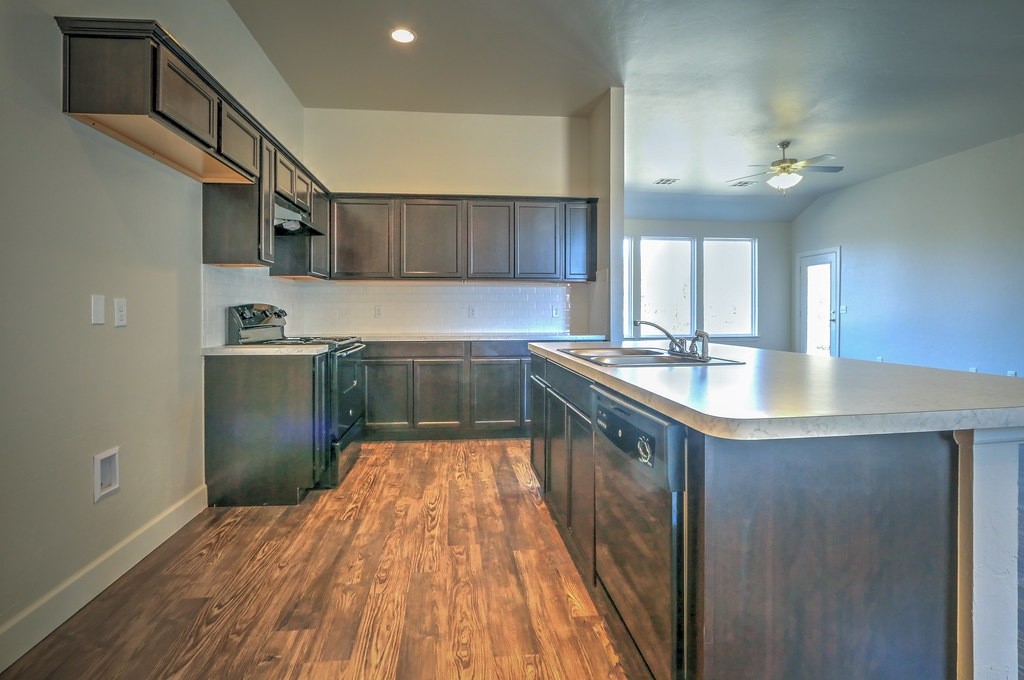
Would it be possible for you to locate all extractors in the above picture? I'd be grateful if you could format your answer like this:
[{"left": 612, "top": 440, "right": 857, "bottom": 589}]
[{"left": 274, "top": 194, "right": 325, "bottom": 238}]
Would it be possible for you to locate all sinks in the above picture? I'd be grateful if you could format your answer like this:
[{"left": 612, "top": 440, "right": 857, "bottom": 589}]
[
  {"left": 587, "top": 357, "right": 746, "bottom": 366},
  {"left": 557, "top": 348, "right": 664, "bottom": 356}
]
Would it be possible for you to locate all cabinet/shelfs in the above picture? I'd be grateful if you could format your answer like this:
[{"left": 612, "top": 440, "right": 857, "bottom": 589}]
[
  {"left": 56, "top": 17, "right": 599, "bottom": 281},
  {"left": 204, "top": 341, "right": 593, "bottom": 600}
]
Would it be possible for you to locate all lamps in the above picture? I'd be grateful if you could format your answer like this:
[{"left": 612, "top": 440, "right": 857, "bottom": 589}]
[{"left": 766, "top": 170, "right": 803, "bottom": 196}]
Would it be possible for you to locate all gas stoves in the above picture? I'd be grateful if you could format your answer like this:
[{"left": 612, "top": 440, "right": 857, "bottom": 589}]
[{"left": 226, "top": 302, "right": 364, "bottom": 353}]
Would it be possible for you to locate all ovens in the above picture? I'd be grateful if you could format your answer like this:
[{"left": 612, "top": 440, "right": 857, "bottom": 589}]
[{"left": 322, "top": 342, "right": 366, "bottom": 491}]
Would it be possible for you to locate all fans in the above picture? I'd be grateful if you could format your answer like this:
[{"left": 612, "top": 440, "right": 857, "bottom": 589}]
[{"left": 725, "top": 142, "right": 844, "bottom": 183}]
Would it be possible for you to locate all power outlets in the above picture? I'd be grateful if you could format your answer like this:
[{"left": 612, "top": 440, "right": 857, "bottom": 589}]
[
  {"left": 552, "top": 305, "right": 559, "bottom": 317},
  {"left": 374, "top": 305, "right": 382, "bottom": 318},
  {"left": 468, "top": 305, "right": 476, "bottom": 317}
]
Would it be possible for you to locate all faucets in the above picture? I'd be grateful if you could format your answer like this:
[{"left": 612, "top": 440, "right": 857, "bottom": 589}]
[
  {"left": 633, "top": 320, "right": 686, "bottom": 355},
  {"left": 695, "top": 330, "right": 710, "bottom": 360}
]
